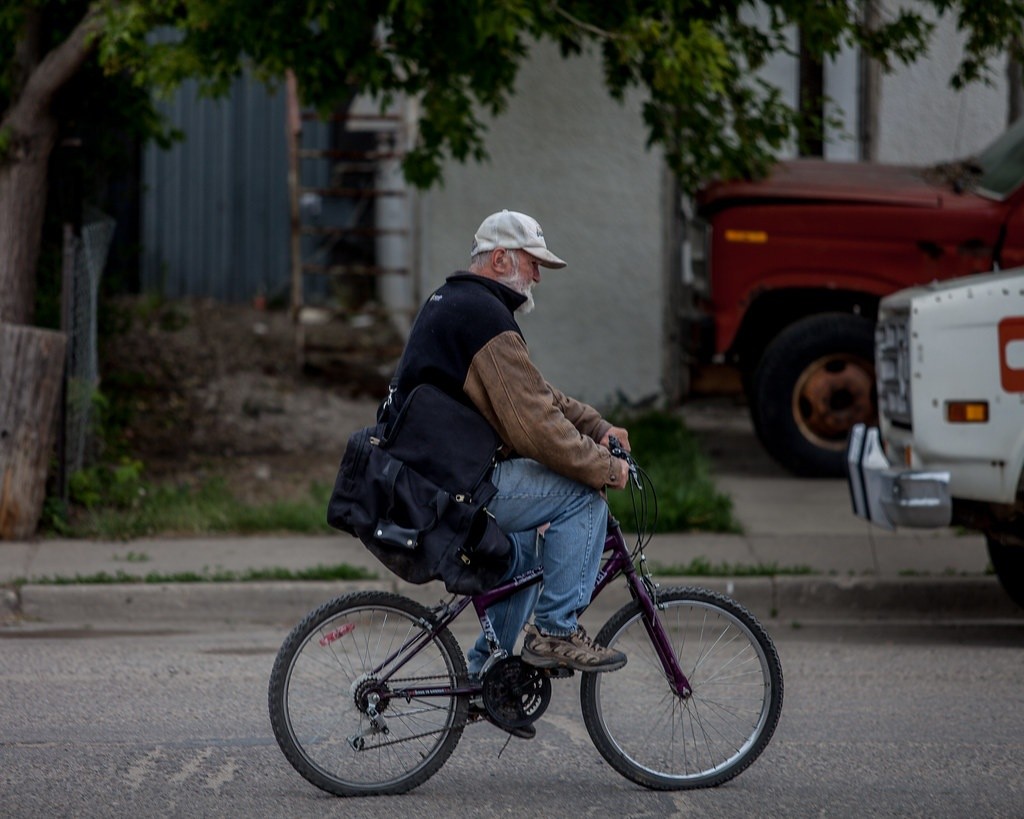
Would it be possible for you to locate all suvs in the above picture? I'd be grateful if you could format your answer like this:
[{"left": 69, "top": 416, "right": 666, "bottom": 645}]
[{"left": 693, "top": 112, "right": 1024, "bottom": 481}]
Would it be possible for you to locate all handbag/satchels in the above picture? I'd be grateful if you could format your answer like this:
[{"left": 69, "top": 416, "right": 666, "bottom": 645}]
[{"left": 326, "top": 386, "right": 513, "bottom": 595}]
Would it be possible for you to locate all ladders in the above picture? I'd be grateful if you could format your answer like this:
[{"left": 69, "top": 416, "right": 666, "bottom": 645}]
[{"left": 282, "top": 33, "right": 427, "bottom": 387}]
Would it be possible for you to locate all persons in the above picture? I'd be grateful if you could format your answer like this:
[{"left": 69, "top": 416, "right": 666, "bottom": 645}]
[{"left": 376, "top": 211, "right": 632, "bottom": 739}]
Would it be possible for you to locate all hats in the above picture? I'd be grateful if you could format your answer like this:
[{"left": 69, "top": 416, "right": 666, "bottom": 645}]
[{"left": 471, "top": 209, "right": 568, "bottom": 269}]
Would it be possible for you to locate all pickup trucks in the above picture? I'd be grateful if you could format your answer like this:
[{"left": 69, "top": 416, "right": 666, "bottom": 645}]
[{"left": 842, "top": 273, "right": 1024, "bottom": 620}]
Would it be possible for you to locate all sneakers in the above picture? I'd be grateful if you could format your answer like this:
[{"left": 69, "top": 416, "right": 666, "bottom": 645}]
[
  {"left": 520, "top": 621, "right": 626, "bottom": 671},
  {"left": 465, "top": 669, "right": 536, "bottom": 740}
]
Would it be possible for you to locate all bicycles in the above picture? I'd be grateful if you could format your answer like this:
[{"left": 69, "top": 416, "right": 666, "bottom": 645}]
[{"left": 268, "top": 435, "right": 786, "bottom": 797}]
